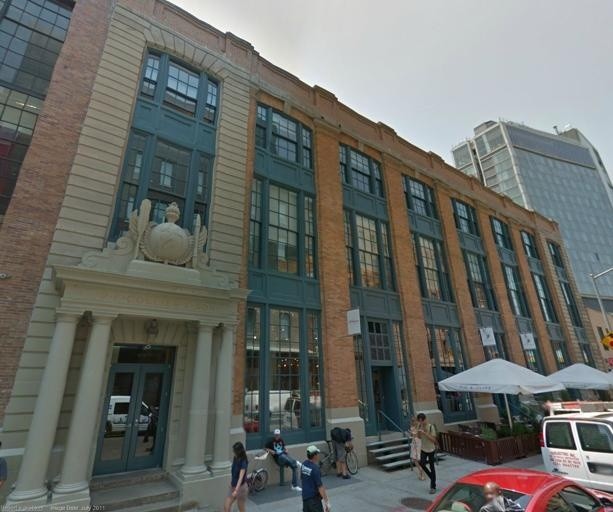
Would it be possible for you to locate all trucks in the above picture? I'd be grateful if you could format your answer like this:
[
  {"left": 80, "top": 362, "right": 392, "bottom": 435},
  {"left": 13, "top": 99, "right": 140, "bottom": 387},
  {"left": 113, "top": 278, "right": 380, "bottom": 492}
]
[
  {"left": 544, "top": 400, "right": 612, "bottom": 416},
  {"left": 243, "top": 387, "right": 291, "bottom": 421},
  {"left": 281, "top": 390, "right": 321, "bottom": 433},
  {"left": 538, "top": 400, "right": 612, "bottom": 494}
]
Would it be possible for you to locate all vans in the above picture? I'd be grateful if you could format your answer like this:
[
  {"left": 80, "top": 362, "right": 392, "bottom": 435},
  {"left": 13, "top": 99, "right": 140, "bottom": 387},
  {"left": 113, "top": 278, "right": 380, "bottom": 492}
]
[{"left": 102, "top": 394, "right": 159, "bottom": 439}]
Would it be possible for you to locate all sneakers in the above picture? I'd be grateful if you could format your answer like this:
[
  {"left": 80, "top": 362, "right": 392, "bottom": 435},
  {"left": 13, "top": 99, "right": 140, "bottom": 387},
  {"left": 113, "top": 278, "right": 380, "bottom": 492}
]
[
  {"left": 296, "top": 460, "right": 302, "bottom": 468},
  {"left": 343, "top": 475, "right": 351, "bottom": 479},
  {"left": 430, "top": 489, "right": 435, "bottom": 494},
  {"left": 337, "top": 472, "right": 342, "bottom": 477},
  {"left": 292, "top": 485, "right": 302, "bottom": 492}
]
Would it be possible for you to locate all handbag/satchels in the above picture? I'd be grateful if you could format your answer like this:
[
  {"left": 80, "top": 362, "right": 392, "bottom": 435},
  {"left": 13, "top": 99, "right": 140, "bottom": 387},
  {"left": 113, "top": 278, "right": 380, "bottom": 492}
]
[{"left": 331, "top": 427, "right": 349, "bottom": 444}]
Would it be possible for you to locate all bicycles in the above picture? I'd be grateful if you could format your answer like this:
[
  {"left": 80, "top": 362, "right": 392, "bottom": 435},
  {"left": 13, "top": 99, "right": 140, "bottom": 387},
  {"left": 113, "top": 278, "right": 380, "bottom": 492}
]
[
  {"left": 246, "top": 454, "right": 268, "bottom": 496},
  {"left": 316, "top": 437, "right": 358, "bottom": 477}
]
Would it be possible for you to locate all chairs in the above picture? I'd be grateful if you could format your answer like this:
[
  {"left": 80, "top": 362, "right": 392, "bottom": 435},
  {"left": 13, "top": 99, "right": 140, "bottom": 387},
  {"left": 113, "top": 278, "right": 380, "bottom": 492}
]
[{"left": 449, "top": 421, "right": 509, "bottom": 462}]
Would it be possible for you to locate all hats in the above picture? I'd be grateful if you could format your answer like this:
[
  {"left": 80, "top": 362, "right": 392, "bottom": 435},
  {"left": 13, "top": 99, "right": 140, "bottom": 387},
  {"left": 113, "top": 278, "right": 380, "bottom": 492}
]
[
  {"left": 274, "top": 429, "right": 280, "bottom": 435},
  {"left": 306, "top": 445, "right": 320, "bottom": 455}
]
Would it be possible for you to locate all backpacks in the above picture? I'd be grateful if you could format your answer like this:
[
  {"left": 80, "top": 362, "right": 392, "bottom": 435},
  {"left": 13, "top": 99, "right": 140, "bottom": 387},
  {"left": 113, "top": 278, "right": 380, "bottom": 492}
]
[{"left": 419, "top": 423, "right": 439, "bottom": 446}]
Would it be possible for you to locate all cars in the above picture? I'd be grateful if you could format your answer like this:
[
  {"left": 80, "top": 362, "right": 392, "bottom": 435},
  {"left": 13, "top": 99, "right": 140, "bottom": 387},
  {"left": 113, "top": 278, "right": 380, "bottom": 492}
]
[
  {"left": 424, "top": 467, "right": 612, "bottom": 511},
  {"left": 436, "top": 393, "right": 463, "bottom": 414},
  {"left": 243, "top": 416, "right": 259, "bottom": 433}
]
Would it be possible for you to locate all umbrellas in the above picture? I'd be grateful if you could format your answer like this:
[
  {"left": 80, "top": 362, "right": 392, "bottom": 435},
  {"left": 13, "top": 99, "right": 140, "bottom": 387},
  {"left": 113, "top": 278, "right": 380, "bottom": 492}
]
[
  {"left": 438, "top": 357, "right": 565, "bottom": 428},
  {"left": 547, "top": 362, "right": 613, "bottom": 390},
  {"left": 607, "top": 369, "right": 613, "bottom": 386}
]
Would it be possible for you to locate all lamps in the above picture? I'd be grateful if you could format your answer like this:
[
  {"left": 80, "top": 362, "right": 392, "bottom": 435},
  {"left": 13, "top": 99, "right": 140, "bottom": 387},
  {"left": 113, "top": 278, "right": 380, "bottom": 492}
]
[{"left": 146, "top": 319, "right": 160, "bottom": 347}]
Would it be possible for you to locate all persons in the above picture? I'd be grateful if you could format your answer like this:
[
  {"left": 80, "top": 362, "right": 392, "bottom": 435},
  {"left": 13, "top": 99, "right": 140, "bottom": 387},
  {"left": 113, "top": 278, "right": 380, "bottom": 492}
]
[
  {"left": 224, "top": 441, "right": 249, "bottom": 512},
  {"left": 332, "top": 428, "right": 353, "bottom": 480},
  {"left": 408, "top": 417, "right": 428, "bottom": 480},
  {"left": 301, "top": 446, "right": 327, "bottom": 511},
  {"left": 265, "top": 430, "right": 302, "bottom": 492},
  {"left": 417, "top": 413, "right": 437, "bottom": 494}
]
